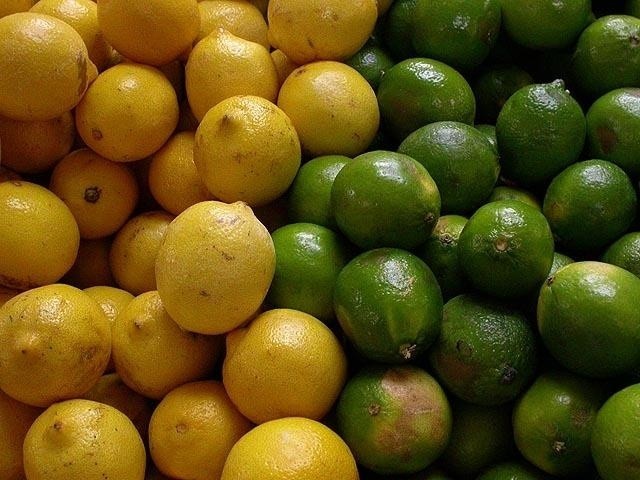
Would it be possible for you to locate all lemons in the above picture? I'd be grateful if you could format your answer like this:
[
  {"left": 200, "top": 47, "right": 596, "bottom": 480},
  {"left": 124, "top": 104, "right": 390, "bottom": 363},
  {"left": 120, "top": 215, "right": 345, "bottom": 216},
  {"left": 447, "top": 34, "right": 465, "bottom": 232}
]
[
  {"left": 269, "top": 0, "right": 640, "bottom": 479},
  {"left": 0, "top": 0, "right": 378, "bottom": 480}
]
